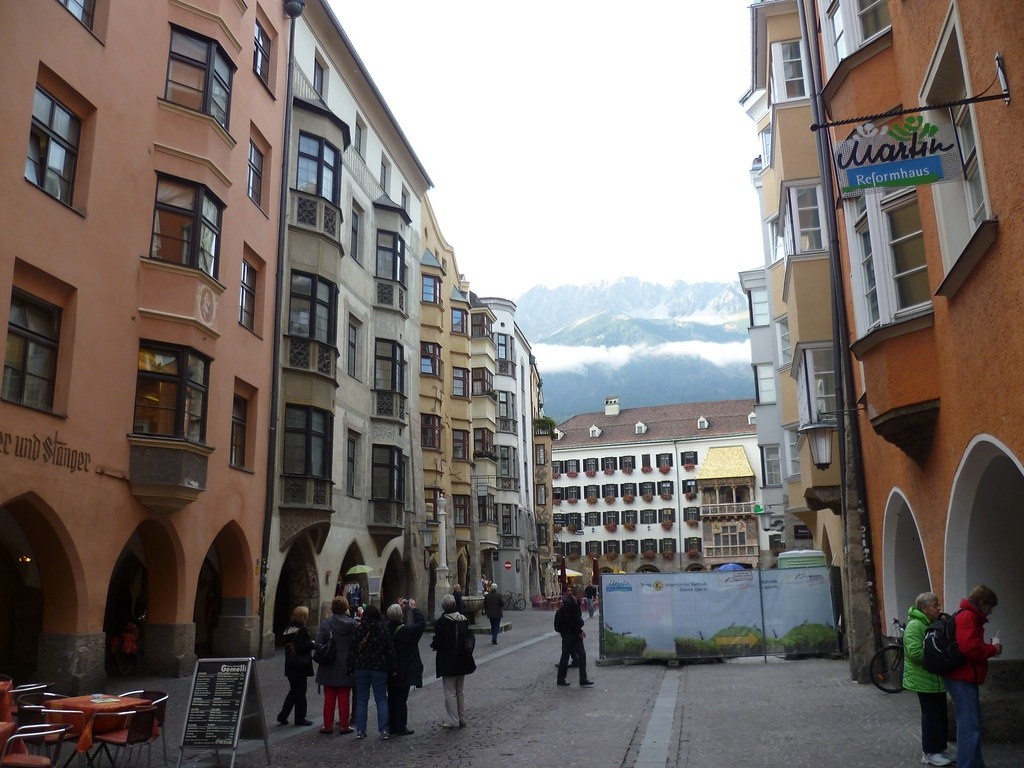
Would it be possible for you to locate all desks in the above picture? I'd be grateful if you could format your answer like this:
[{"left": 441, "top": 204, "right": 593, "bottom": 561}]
[{"left": 43, "top": 693, "right": 154, "bottom": 767}]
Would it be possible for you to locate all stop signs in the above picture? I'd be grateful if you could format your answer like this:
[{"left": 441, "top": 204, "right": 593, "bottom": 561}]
[{"left": 505, "top": 561, "right": 512, "bottom": 570}]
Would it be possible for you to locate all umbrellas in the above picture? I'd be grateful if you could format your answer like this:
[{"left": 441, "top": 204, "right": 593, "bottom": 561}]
[
  {"left": 553, "top": 568, "right": 582, "bottom": 593},
  {"left": 346, "top": 565, "right": 373, "bottom": 574},
  {"left": 719, "top": 563, "right": 744, "bottom": 570}
]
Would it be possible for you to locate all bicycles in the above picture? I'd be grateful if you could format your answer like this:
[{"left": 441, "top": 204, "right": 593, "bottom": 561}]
[
  {"left": 869, "top": 618, "right": 906, "bottom": 693},
  {"left": 503, "top": 591, "right": 526, "bottom": 611}
]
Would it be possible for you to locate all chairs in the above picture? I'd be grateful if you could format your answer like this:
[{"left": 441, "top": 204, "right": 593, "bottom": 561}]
[
  {"left": 529, "top": 593, "right": 590, "bottom": 612},
  {"left": 0, "top": 683, "right": 169, "bottom": 767}
]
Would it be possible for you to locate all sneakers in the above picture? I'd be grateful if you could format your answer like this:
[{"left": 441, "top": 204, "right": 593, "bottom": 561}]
[
  {"left": 941, "top": 750, "right": 956, "bottom": 762},
  {"left": 380, "top": 729, "right": 390, "bottom": 740},
  {"left": 356, "top": 730, "right": 367, "bottom": 739},
  {"left": 922, "top": 749, "right": 950, "bottom": 766}
]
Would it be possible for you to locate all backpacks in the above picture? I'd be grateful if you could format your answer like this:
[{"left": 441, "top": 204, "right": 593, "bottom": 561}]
[{"left": 922, "top": 607, "right": 980, "bottom": 675}]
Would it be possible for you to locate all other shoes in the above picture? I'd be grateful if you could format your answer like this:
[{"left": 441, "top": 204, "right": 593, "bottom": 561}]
[
  {"left": 389, "top": 727, "right": 414, "bottom": 735},
  {"left": 340, "top": 725, "right": 354, "bottom": 733},
  {"left": 295, "top": 719, "right": 313, "bottom": 726},
  {"left": 557, "top": 679, "right": 570, "bottom": 686},
  {"left": 555, "top": 663, "right": 559, "bottom": 667},
  {"left": 580, "top": 680, "right": 593, "bottom": 685},
  {"left": 320, "top": 726, "right": 332, "bottom": 734},
  {"left": 569, "top": 660, "right": 579, "bottom": 668},
  {"left": 277, "top": 716, "right": 288, "bottom": 724},
  {"left": 440, "top": 720, "right": 466, "bottom": 729}
]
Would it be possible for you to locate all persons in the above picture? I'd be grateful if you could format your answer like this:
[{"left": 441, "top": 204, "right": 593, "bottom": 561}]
[
  {"left": 482, "top": 573, "right": 505, "bottom": 644},
  {"left": 453, "top": 584, "right": 464, "bottom": 613},
  {"left": 555, "top": 582, "right": 594, "bottom": 685},
  {"left": 903, "top": 593, "right": 956, "bottom": 768},
  {"left": 941, "top": 585, "right": 1003, "bottom": 768},
  {"left": 276, "top": 595, "right": 426, "bottom": 740},
  {"left": 430, "top": 595, "right": 468, "bottom": 728}
]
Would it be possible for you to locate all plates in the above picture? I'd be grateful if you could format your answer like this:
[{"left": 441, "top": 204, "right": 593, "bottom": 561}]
[{"left": 90, "top": 698, "right": 105, "bottom": 703}]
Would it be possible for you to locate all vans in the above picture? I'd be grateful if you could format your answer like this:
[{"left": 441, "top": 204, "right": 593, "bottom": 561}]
[{"left": 777, "top": 551, "right": 828, "bottom": 569}]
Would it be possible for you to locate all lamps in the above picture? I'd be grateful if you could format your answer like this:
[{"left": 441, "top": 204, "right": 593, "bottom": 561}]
[{"left": 799, "top": 407, "right": 864, "bottom": 472}]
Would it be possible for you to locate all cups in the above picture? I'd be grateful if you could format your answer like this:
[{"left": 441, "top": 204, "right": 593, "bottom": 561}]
[
  {"left": 92, "top": 694, "right": 103, "bottom": 700},
  {"left": 990, "top": 637, "right": 1001, "bottom": 658}
]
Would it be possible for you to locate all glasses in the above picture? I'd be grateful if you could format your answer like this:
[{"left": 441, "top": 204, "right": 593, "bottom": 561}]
[{"left": 923, "top": 604, "right": 941, "bottom": 610}]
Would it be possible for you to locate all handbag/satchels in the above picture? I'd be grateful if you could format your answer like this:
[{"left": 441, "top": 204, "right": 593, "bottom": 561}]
[
  {"left": 110, "top": 632, "right": 139, "bottom": 655},
  {"left": 313, "top": 619, "right": 332, "bottom": 664},
  {"left": 554, "top": 609, "right": 575, "bottom": 635}
]
